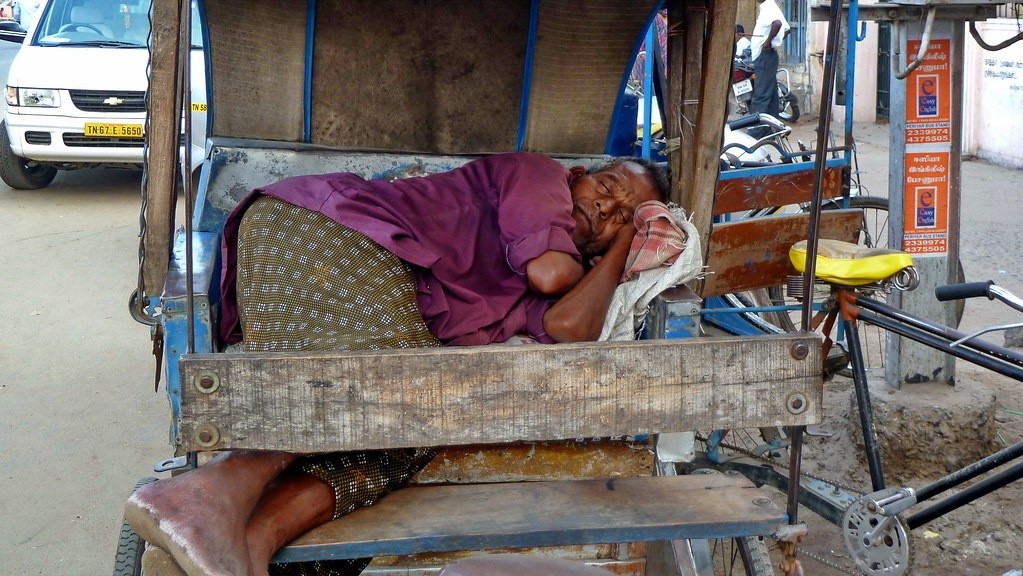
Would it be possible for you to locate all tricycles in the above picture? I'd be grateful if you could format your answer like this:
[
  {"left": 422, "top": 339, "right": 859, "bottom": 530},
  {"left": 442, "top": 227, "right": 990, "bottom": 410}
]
[
  {"left": 107, "top": 0, "right": 838, "bottom": 575},
  {"left": 618, "top": 0, "right": 1023, "bottom": 576}
]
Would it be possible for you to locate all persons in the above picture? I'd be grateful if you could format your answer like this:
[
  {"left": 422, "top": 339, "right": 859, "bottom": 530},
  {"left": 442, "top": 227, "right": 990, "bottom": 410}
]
[
  {"left": 747, "top": 0, "right": 790, "bottom": 140},
  {"left": 126, "top": 151, "right": 670, "bottom": 575},
  {"left": 735, "top": 25, "right": 750, "bottom": 61}
]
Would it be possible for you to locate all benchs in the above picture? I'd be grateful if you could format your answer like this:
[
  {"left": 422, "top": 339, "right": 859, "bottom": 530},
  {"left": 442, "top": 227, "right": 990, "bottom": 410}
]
[{"left": 161, "top": 138, "right": 705, "bottom": 464}]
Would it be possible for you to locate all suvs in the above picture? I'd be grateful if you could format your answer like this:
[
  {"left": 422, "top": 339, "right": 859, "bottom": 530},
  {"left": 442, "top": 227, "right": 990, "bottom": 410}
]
[{"left": 0, "top": 0, "right": 208, "bottom": 192}]
